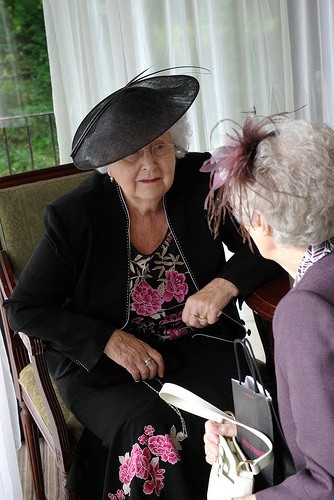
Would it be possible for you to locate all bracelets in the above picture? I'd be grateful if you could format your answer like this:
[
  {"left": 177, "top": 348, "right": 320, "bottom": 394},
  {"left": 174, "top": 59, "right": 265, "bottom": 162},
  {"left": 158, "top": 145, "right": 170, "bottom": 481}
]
[{"left": 225, "top": 411, "right": 235, "bottom": 419}]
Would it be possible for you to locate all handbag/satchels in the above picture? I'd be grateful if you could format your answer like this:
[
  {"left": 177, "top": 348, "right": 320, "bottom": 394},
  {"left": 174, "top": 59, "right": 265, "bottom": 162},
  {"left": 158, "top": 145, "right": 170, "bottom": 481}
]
[
  {"left": 159, "top": 383, "right": 274, "bottom": 500},
  {"left": 231, "top": 338, "right": 296, "bottom": 487}
]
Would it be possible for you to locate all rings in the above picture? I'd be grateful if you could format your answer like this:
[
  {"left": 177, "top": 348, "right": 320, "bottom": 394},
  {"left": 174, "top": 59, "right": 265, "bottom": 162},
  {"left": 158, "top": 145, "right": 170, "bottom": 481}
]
[
  {"left": 144, "top": 357, "right": 154, "bottom": 367},
  {"left": 199, "top": 317, "right": 207, "bottom": 324},
  {"left": 190, "top": 313, "right": 197, "bottom": 320}
]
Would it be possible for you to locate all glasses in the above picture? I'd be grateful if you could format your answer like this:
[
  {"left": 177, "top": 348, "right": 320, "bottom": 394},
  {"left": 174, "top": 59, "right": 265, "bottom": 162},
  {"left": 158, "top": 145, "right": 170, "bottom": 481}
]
[{"left": 124, "top": 144, "right": 175, "bottom": 163}]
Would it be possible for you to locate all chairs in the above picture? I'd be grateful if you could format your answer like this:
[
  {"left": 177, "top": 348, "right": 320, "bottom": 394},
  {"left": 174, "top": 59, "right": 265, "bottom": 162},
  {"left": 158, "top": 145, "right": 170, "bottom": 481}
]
[{"left": 0, "top": 158, "right": 293, "bottom": 500}]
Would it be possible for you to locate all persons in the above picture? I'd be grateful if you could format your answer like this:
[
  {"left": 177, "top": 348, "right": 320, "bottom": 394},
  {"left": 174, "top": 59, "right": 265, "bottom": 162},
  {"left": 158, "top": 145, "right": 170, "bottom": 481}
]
[
  {"left": 2, "top": 65, "right": 284, "bottom": 500},
  {"left": 200, "top": 106, "right": 334, "bottom": 500}
]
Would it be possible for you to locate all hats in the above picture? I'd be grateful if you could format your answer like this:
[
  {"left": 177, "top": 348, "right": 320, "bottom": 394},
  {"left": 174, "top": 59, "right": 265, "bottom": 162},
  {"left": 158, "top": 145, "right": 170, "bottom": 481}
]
[{"left": 69, "top": 66, "right": 211, "bottom": 170}]
